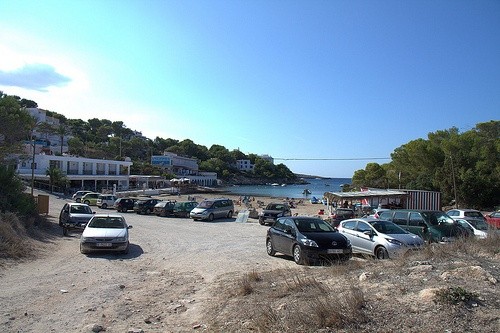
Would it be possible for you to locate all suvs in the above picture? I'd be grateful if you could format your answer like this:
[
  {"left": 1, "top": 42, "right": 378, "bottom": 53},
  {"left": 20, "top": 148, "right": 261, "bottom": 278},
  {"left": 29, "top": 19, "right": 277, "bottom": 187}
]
[
  {"left": 375, "top": 209, "right": 469, "bottom": 245},
  {"left": 190, "top": 197, "right": 235, "bottom": 222},
  {"left": 258, "top": 202, "right": 292, "bottom": 225}
]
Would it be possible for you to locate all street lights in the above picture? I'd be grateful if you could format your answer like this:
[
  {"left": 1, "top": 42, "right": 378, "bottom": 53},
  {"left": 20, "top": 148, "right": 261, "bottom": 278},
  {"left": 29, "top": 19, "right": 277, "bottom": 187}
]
[{"left": 30, "top": 138, "right": 51, "bottom": 196}]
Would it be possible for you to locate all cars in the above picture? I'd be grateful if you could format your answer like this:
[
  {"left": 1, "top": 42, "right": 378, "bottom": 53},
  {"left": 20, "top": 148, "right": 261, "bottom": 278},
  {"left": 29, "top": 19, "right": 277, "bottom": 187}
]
[
  {"left": 331, "top": 208, "right": 358, "bottom": 228},
  {"left": 437, "top": 209, "right": 500, "bottom": 241},
  {"left": 367, "top": 209, "right": 391, "bottom": 219},
  {"left": 79, "top": 214, "right": 133, "bottom": 254},
  {"left": 266, "top": 217, "right": 352, "bottom": 265},
  {"left": 333, "top": 218, "right": 425, "bottom": 260},
  {"left": 59, "top": 203, "right": 96, "bottom": 230},
  {"left": 71, "top": 190, "right": 198, "bottom": 218}
]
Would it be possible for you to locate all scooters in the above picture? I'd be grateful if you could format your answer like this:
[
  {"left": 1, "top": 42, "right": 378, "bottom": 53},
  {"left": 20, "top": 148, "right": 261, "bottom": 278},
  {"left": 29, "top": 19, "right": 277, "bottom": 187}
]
[{"left": 60, "top": 217, "right": 86, "bottom": 236}]
